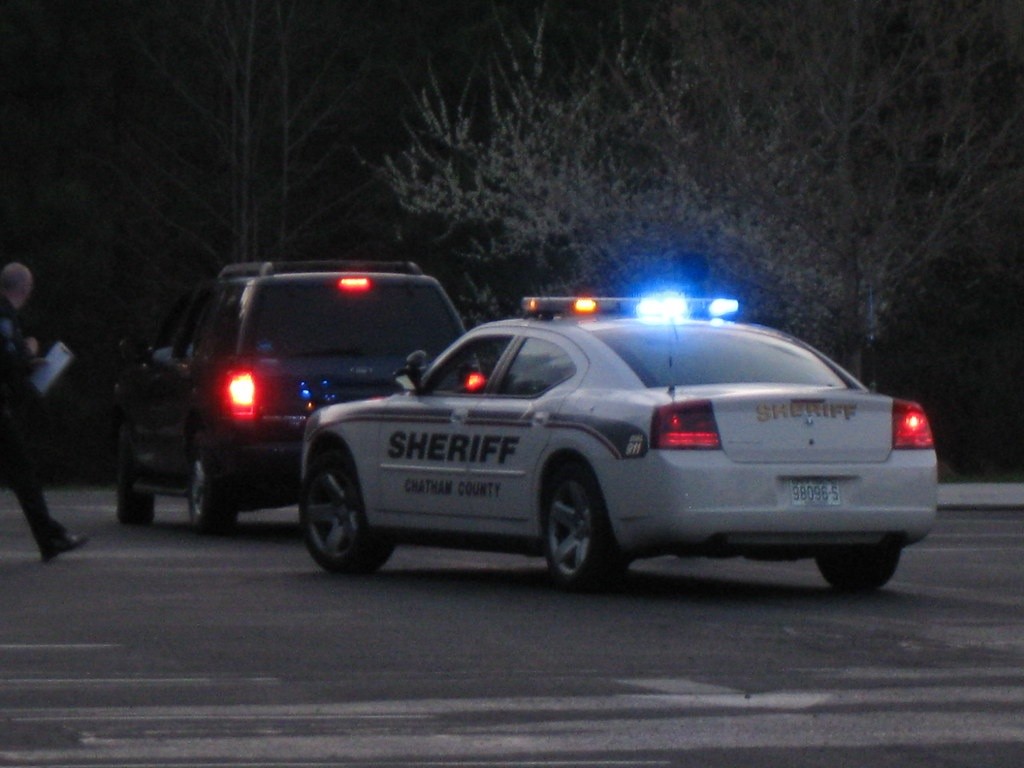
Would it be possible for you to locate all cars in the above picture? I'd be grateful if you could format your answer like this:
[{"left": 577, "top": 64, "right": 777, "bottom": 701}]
[{"left": 300, "top": 295, "right": 938, "bottom": 591}]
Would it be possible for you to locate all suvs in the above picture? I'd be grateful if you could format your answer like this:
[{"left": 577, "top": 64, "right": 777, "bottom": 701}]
[{"left": 115, "top": 261, "right": 484, "bottom": 533}]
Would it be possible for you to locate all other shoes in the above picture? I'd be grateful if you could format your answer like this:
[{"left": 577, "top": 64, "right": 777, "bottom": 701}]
[{"left": 40, "top": 530, "right": 88, "bottom": 561}]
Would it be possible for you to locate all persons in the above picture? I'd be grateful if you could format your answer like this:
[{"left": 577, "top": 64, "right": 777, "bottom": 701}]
[{"left": 0, "top": 262, "right": 88, "bottom": 562}]
